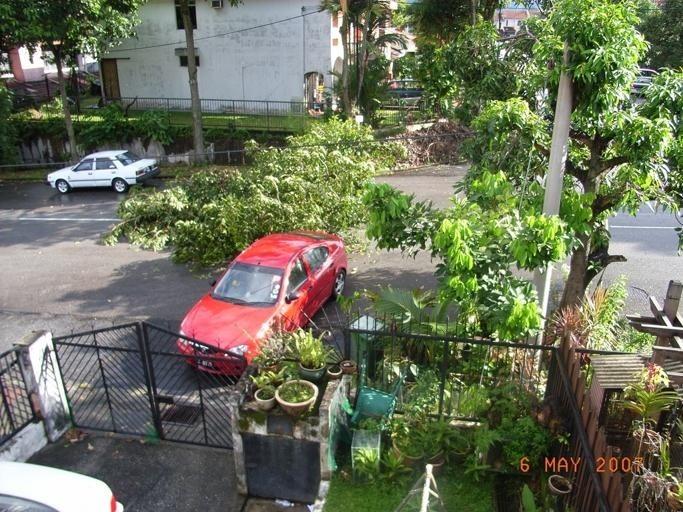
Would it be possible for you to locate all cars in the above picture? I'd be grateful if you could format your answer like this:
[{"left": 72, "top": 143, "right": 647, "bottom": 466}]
[
  {"left": 75, "top": 61, "right": 101, "bottom": 96},
  {"left": 47, "top": 150, "right": 161, "bottom": 194},
  {"left": 0, "top": 461, "right": 124, "bottom": 512},
  {"left": 176, "top": 230, "right": 347, "bottom": 377}
]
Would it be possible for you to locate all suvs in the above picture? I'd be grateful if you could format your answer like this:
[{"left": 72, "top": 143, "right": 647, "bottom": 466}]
[{"left": 368, "top": 79, "right": 431, "bottom": 111}]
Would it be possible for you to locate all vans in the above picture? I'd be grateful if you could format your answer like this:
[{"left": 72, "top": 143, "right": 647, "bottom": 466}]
[{"left": 630, "top": 68, "right": 661, "bottom": 94}]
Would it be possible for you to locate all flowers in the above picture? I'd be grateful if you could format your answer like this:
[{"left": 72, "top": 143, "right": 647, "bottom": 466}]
[{"left": 620, "top": 362, "right": 678, "bottom": 437}]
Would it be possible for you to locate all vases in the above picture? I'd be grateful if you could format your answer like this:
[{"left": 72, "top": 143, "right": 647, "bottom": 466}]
[{"left": 631, "top": 429, "right": 662, "bottom": 455}]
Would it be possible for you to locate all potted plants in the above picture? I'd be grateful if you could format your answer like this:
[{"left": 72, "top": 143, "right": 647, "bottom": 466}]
[
  {"left": 247, "top": 322, "right": 358, "bottom": 421},
  {"left": 387, "top": 408, "right": 576, "bottom": 500}
]
